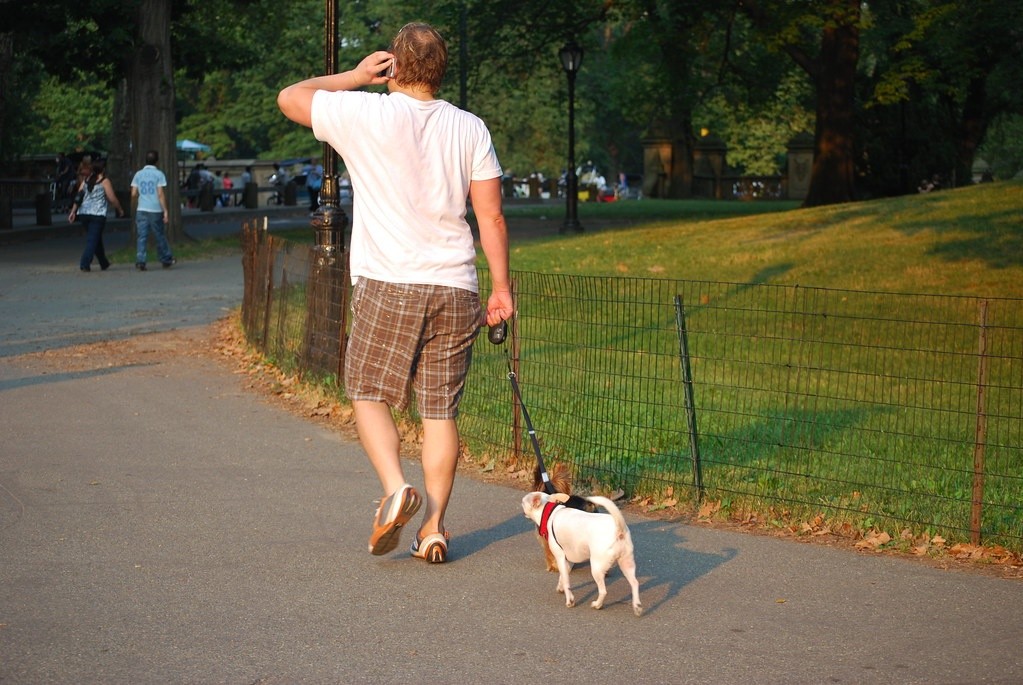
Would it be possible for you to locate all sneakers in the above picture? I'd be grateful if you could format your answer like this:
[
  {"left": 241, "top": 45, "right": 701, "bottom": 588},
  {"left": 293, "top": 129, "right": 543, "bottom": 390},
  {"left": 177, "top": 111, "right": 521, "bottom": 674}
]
[
  {"left": 368, "top": 483, "right": 423, "bottom": 556},
  {"left": 410, "top": 527, "right": 450, "bottom": 563}
]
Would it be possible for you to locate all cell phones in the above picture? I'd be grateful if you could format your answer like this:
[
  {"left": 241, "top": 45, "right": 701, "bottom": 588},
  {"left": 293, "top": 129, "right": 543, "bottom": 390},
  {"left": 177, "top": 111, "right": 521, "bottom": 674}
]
[{"left": 380, "top": 58, "right": 396, "bottom": 77}]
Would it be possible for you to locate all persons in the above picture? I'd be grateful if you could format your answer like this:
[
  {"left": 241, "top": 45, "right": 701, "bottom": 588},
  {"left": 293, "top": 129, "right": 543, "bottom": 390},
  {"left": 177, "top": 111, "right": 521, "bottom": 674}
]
[
  {"left": 181, "top": 162, "right": 233, "bottom": 207},
  {"left": 613, "top": 170, "right": 627, "bottom": 200},
  {"left": 236, "top": 166, "right": 252, "bottom": 207},
  {"left": 75, "top": 155, "right": 92, "bottom": 190},
  {"left": 278, "top": 22, "right": 513, "bottom": 563},
  {"left": 593, "top": 172, "right": 607, "bottom": 203},
  {"left": 273, "top": 163, "right": 297, "bottom": 206},
  {"left": 130, "top": 150, "right": 176, "bottom": 270},
  {"left": 305, "top": 158, "right": 323, "bottom": 212},
  {"left": 69, "top": 158, "right": 125, "bottom": 272}
]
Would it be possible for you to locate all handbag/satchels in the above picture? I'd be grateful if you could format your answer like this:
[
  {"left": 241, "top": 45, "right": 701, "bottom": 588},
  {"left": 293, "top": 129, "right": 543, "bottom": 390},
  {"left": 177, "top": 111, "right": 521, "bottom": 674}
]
[{"left": 74, "top": 190, "right": 85, "bottom": 207}]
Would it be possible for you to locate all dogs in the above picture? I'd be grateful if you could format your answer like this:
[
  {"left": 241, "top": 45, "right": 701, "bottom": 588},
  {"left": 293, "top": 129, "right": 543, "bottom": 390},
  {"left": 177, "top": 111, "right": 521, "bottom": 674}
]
[
  {"left": 534, "top": 463, "right": 600, "bottom": 572},
  {"left": 521, "top": 491, "right": 643, "bottom": 617}
]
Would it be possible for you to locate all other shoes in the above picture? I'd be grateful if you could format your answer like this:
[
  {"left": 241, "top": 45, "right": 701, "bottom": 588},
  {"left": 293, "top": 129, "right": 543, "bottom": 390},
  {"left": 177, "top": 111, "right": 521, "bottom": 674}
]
[
  {"left": 80, "top": 266, "right": 90, "bottom": 272},
  {"left": 162, "top": 258, "right": 176, "bottom": 268},
  {"left": 101, "top": 262, "right": 112, "bottom": 270},
  {"left": 136, "top": 262, "right": 147, "bottom": 271}
]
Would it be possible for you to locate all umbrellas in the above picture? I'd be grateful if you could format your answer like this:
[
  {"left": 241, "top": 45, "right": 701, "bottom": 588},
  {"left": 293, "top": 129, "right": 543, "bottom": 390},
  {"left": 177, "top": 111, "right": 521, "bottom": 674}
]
[{"left": 176, "top": 138, "right": 211, "bottom": 182}]
